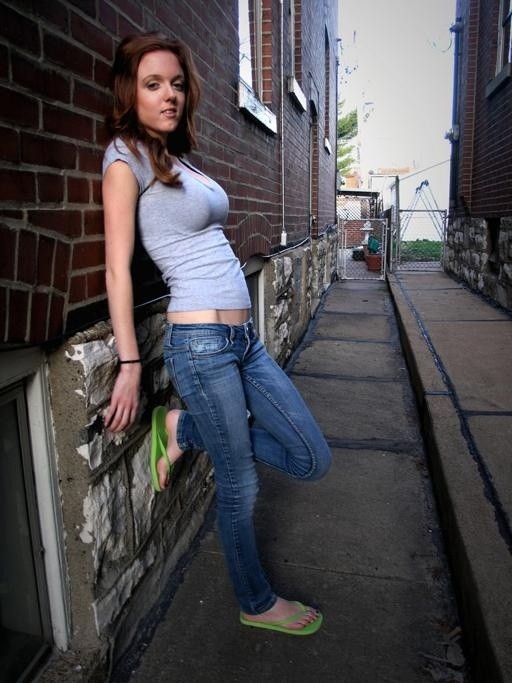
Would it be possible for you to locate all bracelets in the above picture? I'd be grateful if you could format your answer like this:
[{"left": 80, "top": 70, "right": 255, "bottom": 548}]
[{"left": 116, "top": 358, "right": 144, "bottom": 364}]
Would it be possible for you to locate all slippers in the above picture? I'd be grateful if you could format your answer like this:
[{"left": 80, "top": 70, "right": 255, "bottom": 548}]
[
  {"left": 151, "top": 406, "right": 177, "bottom": 493},
  {"left": 240, "top": 600, "right": 323, "bottom": 635}
]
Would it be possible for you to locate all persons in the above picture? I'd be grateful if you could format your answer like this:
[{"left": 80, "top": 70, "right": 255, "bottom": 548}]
[{"left": 101, "top": 29, "right": 333, "bottom": 637}]
